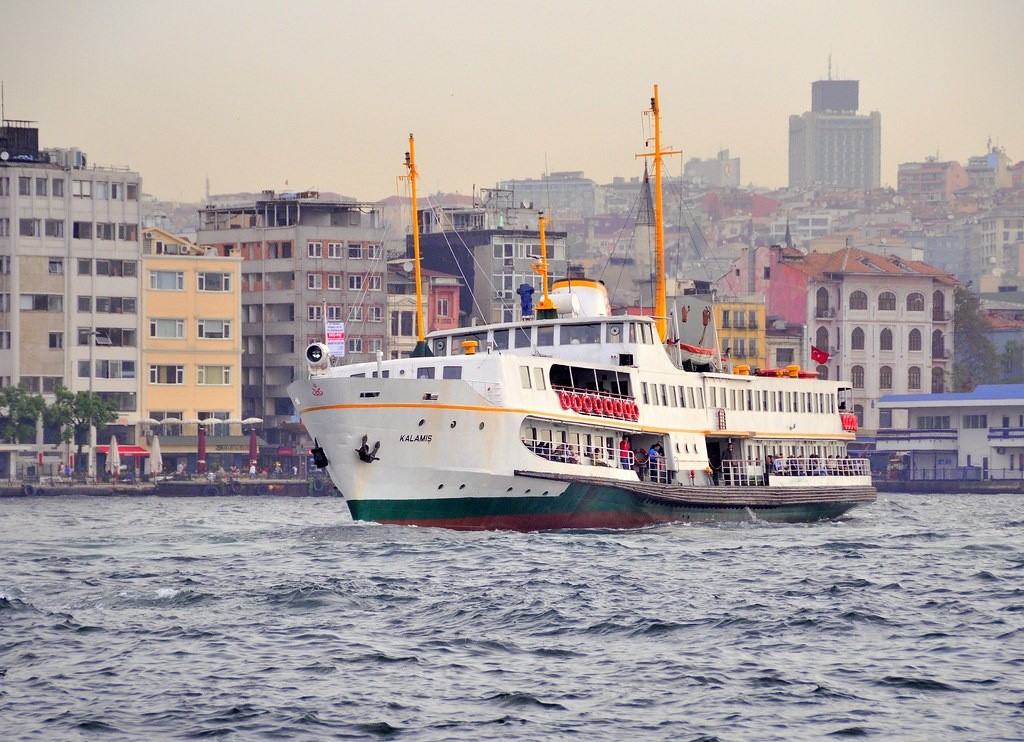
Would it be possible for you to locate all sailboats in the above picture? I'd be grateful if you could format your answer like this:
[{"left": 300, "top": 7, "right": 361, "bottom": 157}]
[{"left": 287, "top": 80, "right": 878, "bottom": 531}]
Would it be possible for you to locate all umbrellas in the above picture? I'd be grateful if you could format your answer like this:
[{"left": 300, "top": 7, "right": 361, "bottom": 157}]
[
  {"left": 196, "top": 426, "right": 206, "bottom": 478},
  {"left": 248, "top": 427, "right": 258, "bottom": 469},
  {"left": 149, "top": 433, "right": 163, "bottom": 486},
  {"left": 105, "top": 436, "right": 121, "bottom": 485},
  {"left": 138, "top": 417, "right": 264, "bottom": 437}
]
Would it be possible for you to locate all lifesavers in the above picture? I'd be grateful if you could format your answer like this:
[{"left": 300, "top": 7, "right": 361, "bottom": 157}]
[
  {"left": 843, "top": 415, "right": 858, "bottom": 430},
  {"left": 560, "top": 393, "right": 571, "bottom": 410},
  {"left": 592, "top": 397, "right": 604, "bottom": 414},
  {"left": 581, "top": 395, "right": 593, "bottom": 412},
  {"left": 570, "top": 394, "right": 583, "bottom": 411},
  {"left": 630, "top": 402, "right": 640, "bottom": 419},
  {"left": 603, "top": 399, "right": 614, "bottom": 415},
  {"left": 622, "top": 402, "right": 631, "bottom": 418},
  {"left": 612, "top": 401, "right": 623, "bottom": 417}
]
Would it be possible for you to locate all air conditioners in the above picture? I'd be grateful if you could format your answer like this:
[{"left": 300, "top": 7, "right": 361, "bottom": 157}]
[
  {"left": 494, "top": 290, "right": 505, "bottom": 298},
  {"left": 180, "top": 245, "right": 188, "bottom": 253},
  {"left": 823, "top": 311, "right": 831, "bottom": 318},
  {"left": 145, "top": 232, "right": 153, "bottom": 239}
]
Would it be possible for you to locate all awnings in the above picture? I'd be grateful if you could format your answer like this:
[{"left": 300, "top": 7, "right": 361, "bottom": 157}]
[{"left": 96, "top": 446, "right": 149, "bottom": 458}]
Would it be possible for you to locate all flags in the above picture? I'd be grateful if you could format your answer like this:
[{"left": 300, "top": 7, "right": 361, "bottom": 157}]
[{"left": 811, "top": 345, "right": 829, "bottom": 364}]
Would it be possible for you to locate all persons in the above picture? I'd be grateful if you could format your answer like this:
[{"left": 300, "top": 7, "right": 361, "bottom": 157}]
[
  {"left": 523, "top": 436, "right": 908, "bottom": 481},
  {"left": 176, "top": 460, "right": 326, "bottom": 481},
  {"left": 58, "top": 462, "right": 141, "bottom": 487}
]
